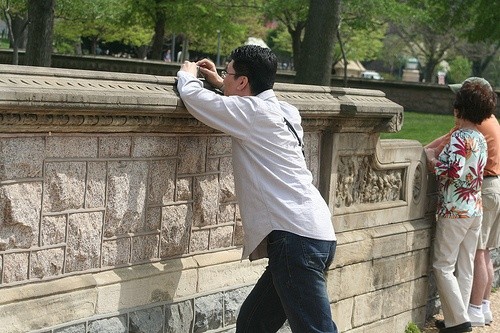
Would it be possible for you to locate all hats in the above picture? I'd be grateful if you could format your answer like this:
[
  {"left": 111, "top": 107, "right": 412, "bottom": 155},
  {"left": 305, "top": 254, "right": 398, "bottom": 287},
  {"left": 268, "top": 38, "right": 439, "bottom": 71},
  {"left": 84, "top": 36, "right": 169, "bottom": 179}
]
[{"left": 448, "top": 77, "right": 493, "bottom": 96}]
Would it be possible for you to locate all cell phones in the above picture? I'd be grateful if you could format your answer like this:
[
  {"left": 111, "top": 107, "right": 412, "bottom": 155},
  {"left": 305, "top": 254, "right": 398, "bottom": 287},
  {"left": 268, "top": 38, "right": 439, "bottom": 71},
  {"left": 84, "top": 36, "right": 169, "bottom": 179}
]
[{"left": 195, "top": 65, "right": 206, "bottom": 78}]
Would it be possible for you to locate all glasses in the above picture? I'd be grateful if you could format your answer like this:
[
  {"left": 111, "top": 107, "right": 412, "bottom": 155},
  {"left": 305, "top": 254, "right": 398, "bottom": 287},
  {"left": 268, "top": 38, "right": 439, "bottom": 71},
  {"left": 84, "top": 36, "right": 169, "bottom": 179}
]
[{"left": 220, "top": 70, "right": 240, "bottom": 79}]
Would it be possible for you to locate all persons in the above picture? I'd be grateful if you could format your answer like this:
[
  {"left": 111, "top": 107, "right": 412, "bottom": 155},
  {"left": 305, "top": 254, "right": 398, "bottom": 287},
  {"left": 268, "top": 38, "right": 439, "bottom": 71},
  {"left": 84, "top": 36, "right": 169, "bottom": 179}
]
[
  {"left": 422, "top": 76, "right": 499, "bottom": 327},
  {"left": 176, "top": 44, "right": 340, "bottom": 333},
  {"left": 423, "top": 82, "right": 488, "bottom": 333}
]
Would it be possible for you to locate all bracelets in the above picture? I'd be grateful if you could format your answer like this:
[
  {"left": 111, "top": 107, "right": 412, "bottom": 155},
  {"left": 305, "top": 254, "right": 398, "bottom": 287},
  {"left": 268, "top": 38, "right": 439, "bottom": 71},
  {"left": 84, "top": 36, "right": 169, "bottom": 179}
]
[{"left": 429, "top": 157, "right": 435, "bottom": 166}]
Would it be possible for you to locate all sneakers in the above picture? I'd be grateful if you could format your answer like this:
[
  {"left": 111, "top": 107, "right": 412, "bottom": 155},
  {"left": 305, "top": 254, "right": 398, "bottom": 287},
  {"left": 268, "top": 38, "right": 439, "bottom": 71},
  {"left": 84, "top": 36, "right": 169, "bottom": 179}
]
[
  {"left": 440, "top": 322, "right": 472, "bottom": 332},
  {"left": 482, "top": 311, "right": 492, "bottom": 325},
  {"left": 435, "top": 320, "right": 445, "bottom": 328},
  {"left": 467, "top": 314, "right": 486, "bottom": 326}
]
